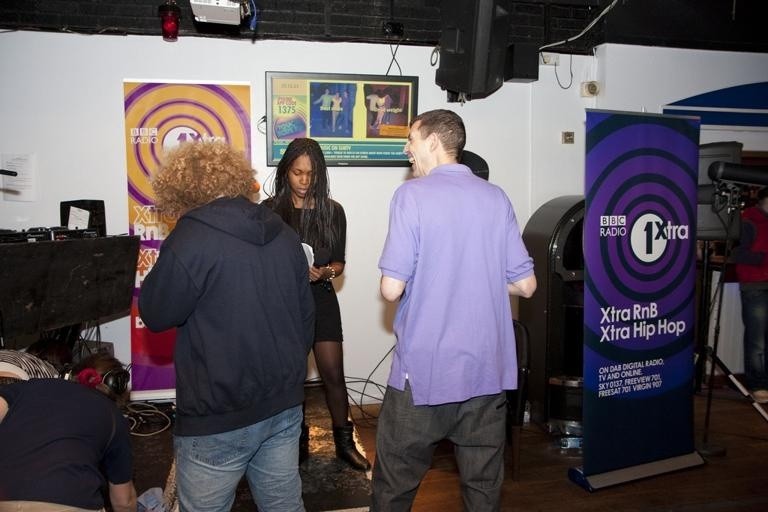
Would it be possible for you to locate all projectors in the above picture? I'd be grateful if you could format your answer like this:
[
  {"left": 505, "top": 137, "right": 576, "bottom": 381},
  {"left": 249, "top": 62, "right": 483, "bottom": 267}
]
[{"left": 189, "top": 0, "right": 251, "bottom": 25}]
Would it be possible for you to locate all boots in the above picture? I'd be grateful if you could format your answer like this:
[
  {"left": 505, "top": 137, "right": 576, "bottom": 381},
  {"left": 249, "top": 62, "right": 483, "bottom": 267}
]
[
  {"left": 299, "top": 424, "right": 310, "bottom": 466},
  {"left": 332, "top": 421, "right": 371, "bottom": 473}
]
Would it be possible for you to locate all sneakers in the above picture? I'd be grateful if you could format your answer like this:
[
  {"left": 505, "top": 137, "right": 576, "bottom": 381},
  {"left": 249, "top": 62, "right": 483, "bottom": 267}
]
[{"left": 748, "top": 387, "right": 768, "bottom": 404}]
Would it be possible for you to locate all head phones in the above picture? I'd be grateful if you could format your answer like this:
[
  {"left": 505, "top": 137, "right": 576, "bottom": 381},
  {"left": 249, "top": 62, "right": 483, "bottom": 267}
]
[{"left": 60, "top": 354, "right": 135, "bottom": 397}]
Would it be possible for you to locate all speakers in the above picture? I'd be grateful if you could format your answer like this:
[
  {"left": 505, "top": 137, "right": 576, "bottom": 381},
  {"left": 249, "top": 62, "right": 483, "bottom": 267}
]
[{"left": 695, "top": 142, "right": 742, "bottom": 240}]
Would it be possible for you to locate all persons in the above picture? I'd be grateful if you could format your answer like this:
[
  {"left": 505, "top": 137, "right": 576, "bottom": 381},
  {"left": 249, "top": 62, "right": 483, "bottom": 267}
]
[
  {"left": 313, "top": 86, "right": 331, "bottom": 129},
  {"left": 339, "top": 89, "right": 351, "bottom": 133},
  {"left": 365, "top": 89, "right": 388, "bottom": 128},
  {"left": 376, "top": 87, "right": 396, "bottom": 125},
  {"left": 258, "top": 137, "right": 371, "bottom": 473},
  {"left": 367, "top": 107, "right": 540, "bottom": 512},
  {"left": 0, "top": 353, "right": 143, "bottom": 512},
  {"left": 330, "top": 90, "right": 341, "bottom": 132},
  {"left": 733, "top": 185, "right": 767, "bottom": 404},
  {"left": 137, "top": 136, "right": 317, "bottom": 512}
]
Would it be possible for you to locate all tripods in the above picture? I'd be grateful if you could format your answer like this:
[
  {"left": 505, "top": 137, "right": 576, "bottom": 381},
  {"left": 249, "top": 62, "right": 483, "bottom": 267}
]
[{"left": 695, "top": 241, "right": 768, "bottom": 426}]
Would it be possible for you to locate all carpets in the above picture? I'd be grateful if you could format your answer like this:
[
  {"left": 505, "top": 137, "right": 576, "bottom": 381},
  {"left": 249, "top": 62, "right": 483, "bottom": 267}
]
[{"left": 121, "top": 381, "right": 375, "bottom": 510}]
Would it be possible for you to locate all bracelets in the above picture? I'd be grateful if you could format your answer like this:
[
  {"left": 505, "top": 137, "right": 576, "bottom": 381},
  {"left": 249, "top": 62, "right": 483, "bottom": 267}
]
[{"left": 321, "top": 265, "right": 335, "bottom": 283}]
